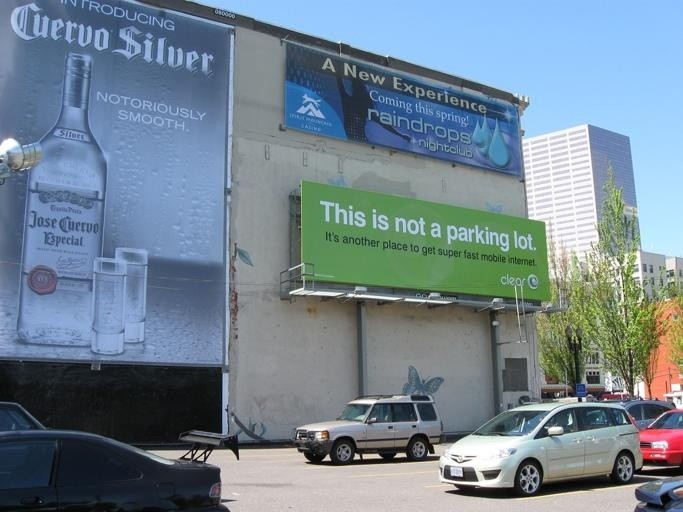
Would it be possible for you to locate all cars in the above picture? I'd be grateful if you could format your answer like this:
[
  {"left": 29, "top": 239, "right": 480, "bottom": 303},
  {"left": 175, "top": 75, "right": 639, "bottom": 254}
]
[{"left": 0, "top": 400, "right": 239, "bottom": 511}]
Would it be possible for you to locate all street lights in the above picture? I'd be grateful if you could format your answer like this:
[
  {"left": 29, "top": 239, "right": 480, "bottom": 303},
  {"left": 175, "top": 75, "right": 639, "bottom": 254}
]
[{"left": 564, "top": 326, "right": 583, "bottom": 403}]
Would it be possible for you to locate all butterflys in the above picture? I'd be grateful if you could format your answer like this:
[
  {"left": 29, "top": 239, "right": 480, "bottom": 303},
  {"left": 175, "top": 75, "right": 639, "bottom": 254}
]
[{"left": 401, "top": 365, "right": 444, "bottom": 394}]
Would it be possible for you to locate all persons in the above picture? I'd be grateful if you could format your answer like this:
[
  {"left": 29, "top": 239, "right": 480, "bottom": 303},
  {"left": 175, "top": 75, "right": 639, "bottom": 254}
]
[{"left": 337, "top": 75, "right": 411, "bottom": 143}]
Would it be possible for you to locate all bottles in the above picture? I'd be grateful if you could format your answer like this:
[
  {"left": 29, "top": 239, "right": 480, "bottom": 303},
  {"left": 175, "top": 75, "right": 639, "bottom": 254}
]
[{"left": 14, "top": 53, "right": 108, "bottom": 351}]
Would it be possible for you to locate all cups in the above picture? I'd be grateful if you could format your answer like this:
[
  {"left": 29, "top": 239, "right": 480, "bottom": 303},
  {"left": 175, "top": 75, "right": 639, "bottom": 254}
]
[{"left": 90, "top": 245, "right": 148, "bottom": 357}]
